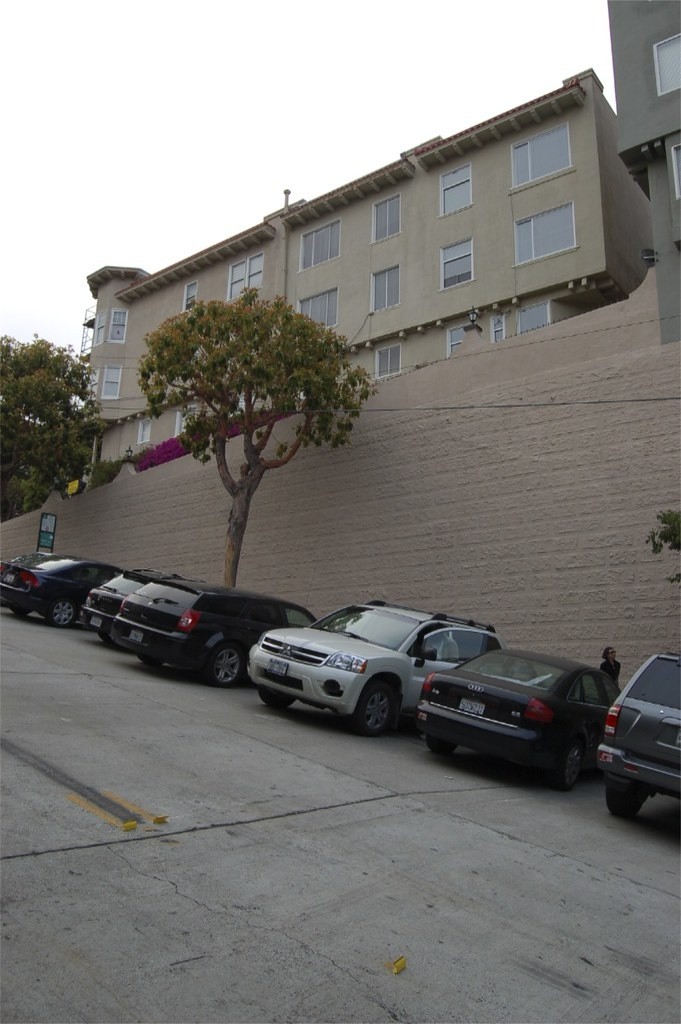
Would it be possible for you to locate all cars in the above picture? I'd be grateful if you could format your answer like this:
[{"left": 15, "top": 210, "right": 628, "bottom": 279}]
[
  {"left": 111, "top": 579, "right": 318, "bottom": 690},
  {"left": 414, "top": 648, "right": 622, "bottom": 793},
  {"left": 0, "top": 551, "right": 125, "bottom": 629},
  {"left": 80, "top": 567, "right": 188, "bottom": 644}
]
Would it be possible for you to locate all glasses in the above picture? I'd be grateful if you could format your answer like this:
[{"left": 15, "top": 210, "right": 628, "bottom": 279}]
[{"left": 609, "top": 651, "right": 616, "bottom": 654}]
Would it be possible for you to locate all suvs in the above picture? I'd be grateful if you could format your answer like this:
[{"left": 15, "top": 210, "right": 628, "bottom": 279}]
[
  {"left": 246, "top": 599, "right": 508, "bottom": 739},
  {"left": 596, "top": 651, "right": 680, "bottom": 819}
]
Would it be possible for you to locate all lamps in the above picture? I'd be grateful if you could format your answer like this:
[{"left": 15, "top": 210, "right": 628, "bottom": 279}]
[
  {"left": 126, "top": 444, "right": 133, "bottom": 459},
  {"left": 468, "top": 307, "right": 480, "bottom": 326}
]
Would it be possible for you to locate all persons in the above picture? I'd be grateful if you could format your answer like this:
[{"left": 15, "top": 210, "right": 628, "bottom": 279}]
[
  {"left": 599, "top": 647, "right": 621, "bottom": 682},
  {"left": 426, "top": 631, "right": 459, "bottom": 660}
]
